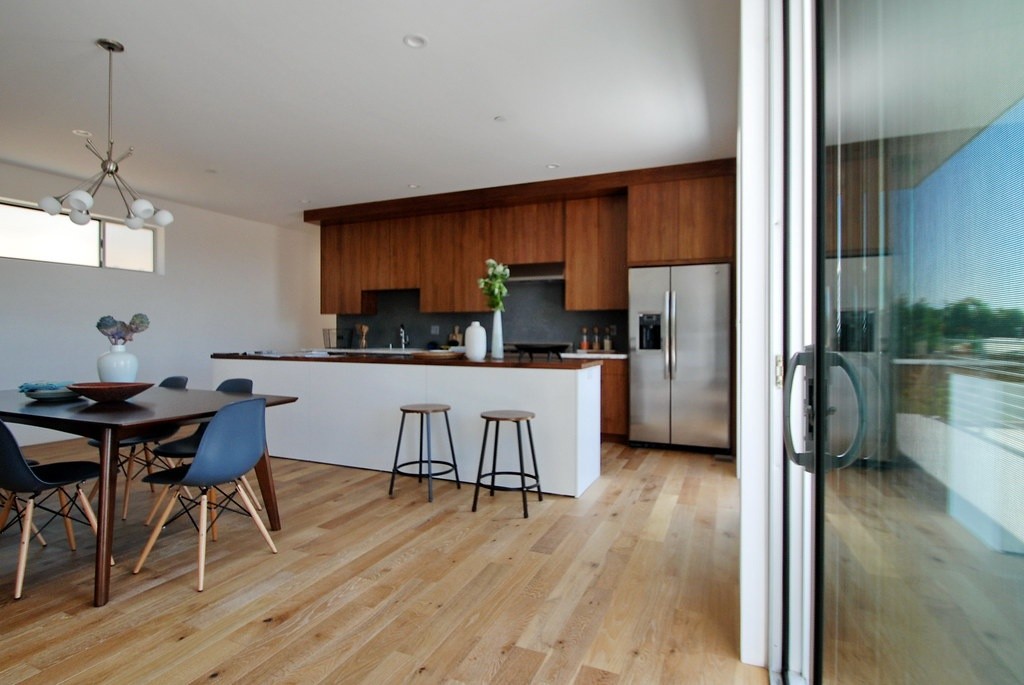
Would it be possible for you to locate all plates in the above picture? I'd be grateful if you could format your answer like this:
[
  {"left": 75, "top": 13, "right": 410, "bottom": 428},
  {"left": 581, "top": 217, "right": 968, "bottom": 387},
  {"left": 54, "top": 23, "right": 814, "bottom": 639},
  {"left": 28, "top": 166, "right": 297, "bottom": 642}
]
[
  {"left": 414, "top": 351, "right": 460, "bottom": 361},
  {"left": 24, "top": 387, "right": 80, "bottom": 402}
]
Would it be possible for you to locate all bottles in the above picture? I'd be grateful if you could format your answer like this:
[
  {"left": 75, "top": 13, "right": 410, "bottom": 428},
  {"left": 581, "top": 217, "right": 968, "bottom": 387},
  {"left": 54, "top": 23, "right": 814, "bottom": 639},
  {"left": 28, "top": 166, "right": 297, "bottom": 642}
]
[
  {"left": 580, "top": 327, "right": 590, "bottom": 350},
  {"left": 603, "top": 328, "right": 612, "bottom": 351},
  {"left": 359, "top": 335, "right": 367, "bottom": 348},
  {"left": 592, "top": 327, "right": 600, "bottom": 350},
  {"left": 464, "top": 320, "right": 486, "bottom": 360}
]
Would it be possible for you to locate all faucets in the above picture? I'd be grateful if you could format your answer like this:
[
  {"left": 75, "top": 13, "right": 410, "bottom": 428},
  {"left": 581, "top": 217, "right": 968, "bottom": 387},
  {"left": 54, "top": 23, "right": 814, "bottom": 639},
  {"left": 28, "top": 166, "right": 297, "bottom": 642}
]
[{"left": 399, "top": 324, "right": 410, "bottom": 348}]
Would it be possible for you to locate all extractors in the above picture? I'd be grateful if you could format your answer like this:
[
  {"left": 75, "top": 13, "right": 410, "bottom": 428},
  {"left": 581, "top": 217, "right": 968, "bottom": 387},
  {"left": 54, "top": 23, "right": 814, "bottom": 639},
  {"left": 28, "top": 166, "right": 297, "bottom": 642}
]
[{"left": 496, "top": 262, "right": 565, "bottom": 280}]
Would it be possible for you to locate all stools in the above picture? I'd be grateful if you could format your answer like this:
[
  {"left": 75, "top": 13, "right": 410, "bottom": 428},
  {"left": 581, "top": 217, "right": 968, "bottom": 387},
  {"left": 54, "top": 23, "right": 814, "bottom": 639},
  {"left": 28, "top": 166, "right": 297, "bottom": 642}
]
[
  {"left": 473, "top": 409, "right": 543, "bottom": 518},
  {"left": 388, "top": 403, "right": 461, "bottom": 502}
]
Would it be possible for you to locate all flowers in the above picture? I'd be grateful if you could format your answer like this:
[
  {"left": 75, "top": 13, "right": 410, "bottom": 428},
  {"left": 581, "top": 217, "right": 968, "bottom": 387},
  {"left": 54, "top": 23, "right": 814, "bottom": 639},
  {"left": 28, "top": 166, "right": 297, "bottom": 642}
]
[{"left": 477, "top": 259, "right": 510, "bottom": 311}]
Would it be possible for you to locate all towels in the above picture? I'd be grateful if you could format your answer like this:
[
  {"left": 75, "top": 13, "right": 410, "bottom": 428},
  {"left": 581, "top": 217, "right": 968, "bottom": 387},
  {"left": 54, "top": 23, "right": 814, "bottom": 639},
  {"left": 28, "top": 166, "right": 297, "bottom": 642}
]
[{"left": 18, "top": 379, "right": 74, "bottom": 393}]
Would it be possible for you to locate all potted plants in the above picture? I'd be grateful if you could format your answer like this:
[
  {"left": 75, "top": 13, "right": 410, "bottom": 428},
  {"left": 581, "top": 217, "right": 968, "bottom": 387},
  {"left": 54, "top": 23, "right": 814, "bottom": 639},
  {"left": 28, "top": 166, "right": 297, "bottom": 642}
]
[{"left": 96, "top": 313, "right": 150, "bottom": 383}]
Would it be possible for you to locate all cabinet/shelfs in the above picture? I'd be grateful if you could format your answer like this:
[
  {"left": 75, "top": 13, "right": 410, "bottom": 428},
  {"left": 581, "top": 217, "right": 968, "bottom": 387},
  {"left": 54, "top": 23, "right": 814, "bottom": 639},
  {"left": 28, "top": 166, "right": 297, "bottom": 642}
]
[{"left": 320, "top": 176, "right": 735, "bottom": 440}]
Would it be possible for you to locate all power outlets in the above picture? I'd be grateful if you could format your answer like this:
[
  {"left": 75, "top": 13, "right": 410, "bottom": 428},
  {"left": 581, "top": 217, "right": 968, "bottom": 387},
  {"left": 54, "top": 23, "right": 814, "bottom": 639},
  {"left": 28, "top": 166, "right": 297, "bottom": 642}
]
[
  {"left": 610, "top": 325, "right": 617, "bottom": 336},
  {"left": 431, "top": 325, "right": 439, "bottom": 335}
]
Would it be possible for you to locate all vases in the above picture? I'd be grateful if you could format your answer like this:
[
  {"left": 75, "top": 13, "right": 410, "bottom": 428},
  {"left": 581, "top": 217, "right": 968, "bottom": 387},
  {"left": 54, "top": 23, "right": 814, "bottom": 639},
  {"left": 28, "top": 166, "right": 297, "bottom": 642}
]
[
  {"left": 491, "top": 308, "right": 505, "bottom": 359},
  {"left": 465, "top": 321, "right": 487, "bottom": 360}
]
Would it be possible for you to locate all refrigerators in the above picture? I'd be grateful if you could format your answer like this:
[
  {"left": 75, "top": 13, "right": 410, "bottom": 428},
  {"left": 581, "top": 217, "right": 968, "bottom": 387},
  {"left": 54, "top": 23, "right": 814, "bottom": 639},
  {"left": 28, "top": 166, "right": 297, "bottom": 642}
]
[{"left": 628, "top": 263, "right": 731, "bottom": 449}]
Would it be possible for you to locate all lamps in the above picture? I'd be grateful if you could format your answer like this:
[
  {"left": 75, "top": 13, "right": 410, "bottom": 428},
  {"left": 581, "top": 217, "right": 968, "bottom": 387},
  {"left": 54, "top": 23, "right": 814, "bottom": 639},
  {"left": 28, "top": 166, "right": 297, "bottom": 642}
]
[{"left": 36, "top": 37, "right": 175, "bottom": 230}]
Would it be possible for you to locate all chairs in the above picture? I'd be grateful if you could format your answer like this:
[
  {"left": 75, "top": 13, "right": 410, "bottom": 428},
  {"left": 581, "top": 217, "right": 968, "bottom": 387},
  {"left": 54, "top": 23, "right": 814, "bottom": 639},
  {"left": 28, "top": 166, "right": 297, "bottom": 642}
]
[
  {"left": 145, "top": 379, "right": 262, "bottom": 541},
  {"left": 0, "top": 459, "right": 48, "bottom": 548},
  {"left": 131, "top": 398, "right": 279, "bottom": 591},
  {"left": 87, "top": 376, "right": 193, "bottom": 521},
  {"left": 0, "top": 419, "right": 116, "bottom": 599}
]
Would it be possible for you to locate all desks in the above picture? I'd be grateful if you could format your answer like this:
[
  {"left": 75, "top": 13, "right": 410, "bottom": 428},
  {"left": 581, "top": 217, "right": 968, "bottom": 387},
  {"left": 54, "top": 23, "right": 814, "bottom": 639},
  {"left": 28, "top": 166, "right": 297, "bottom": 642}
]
[{"left": 0, "top": 385, "right": 298, "bottom": 609}]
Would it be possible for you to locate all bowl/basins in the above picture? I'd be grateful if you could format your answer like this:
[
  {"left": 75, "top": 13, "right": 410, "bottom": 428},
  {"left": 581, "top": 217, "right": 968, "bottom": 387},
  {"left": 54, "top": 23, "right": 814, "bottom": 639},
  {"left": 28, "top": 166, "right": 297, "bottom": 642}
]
[{"left": 65, "top": 382, "right": 155, "bottom": 403}]
